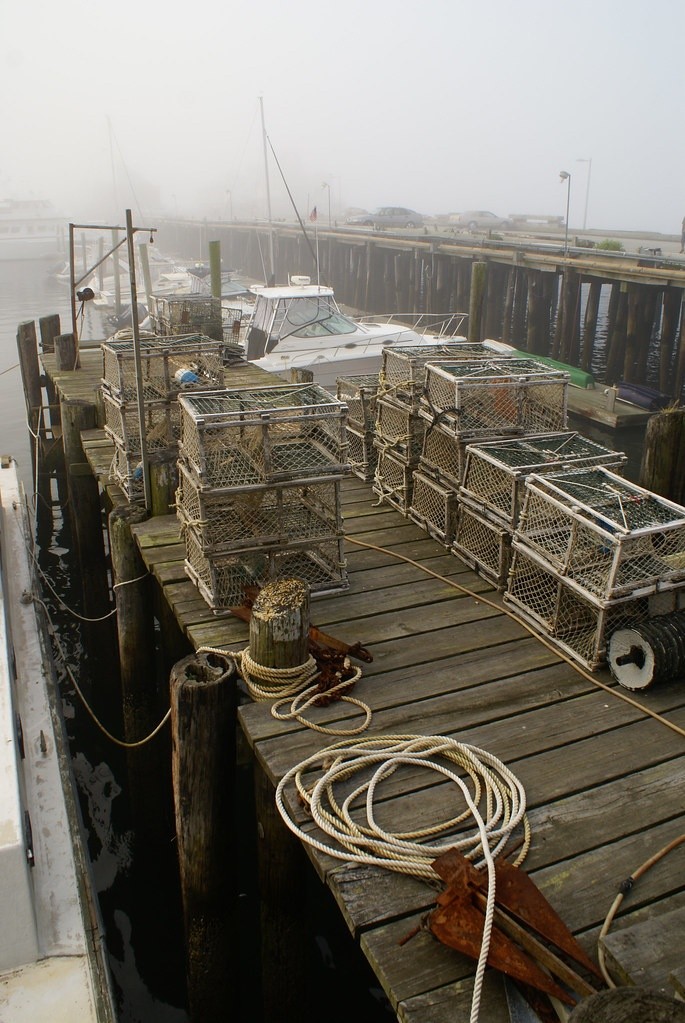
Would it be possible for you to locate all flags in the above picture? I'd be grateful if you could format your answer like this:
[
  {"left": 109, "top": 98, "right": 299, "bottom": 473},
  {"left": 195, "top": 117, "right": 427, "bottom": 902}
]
[{"left": 309, "top": 206, "right": 317, "bottom": 222}]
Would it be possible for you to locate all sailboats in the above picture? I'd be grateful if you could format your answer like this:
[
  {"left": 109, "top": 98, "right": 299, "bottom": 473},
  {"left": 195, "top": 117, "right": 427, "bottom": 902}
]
[{"left": 219, "top": 96, "right": 356, "bottom": 328}]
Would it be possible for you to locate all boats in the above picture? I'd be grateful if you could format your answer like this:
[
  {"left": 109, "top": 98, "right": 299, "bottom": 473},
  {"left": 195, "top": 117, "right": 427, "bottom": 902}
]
[
  {"left": 242, "top": 208, "right": 470, "bottom": 389},
  {"left": 47, "top": 240, "right": 247, "bottom": 306},
  {"left": 106, "top": 291, "right": 258, "bottom": 344},
  {"left": 0, "top": 199, "right": 59, "bottom": 261}
]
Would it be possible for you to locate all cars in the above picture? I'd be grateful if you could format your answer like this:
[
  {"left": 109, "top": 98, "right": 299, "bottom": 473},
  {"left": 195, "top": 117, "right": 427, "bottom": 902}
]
[
  {"left": 448, "top": 211, "right": 511, "bottom": 229},
  {"left": 347, "top": 207, "right": 423, "bottom": 230}
]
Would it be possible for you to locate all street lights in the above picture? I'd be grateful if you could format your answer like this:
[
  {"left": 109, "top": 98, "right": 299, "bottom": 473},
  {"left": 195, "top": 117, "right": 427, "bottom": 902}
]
[
  {"left": 559, "top": 171, "right": 570, "bottom": 257},
  {"left": 320, "top": 183, "right": 331, "bottom": 228}
]
